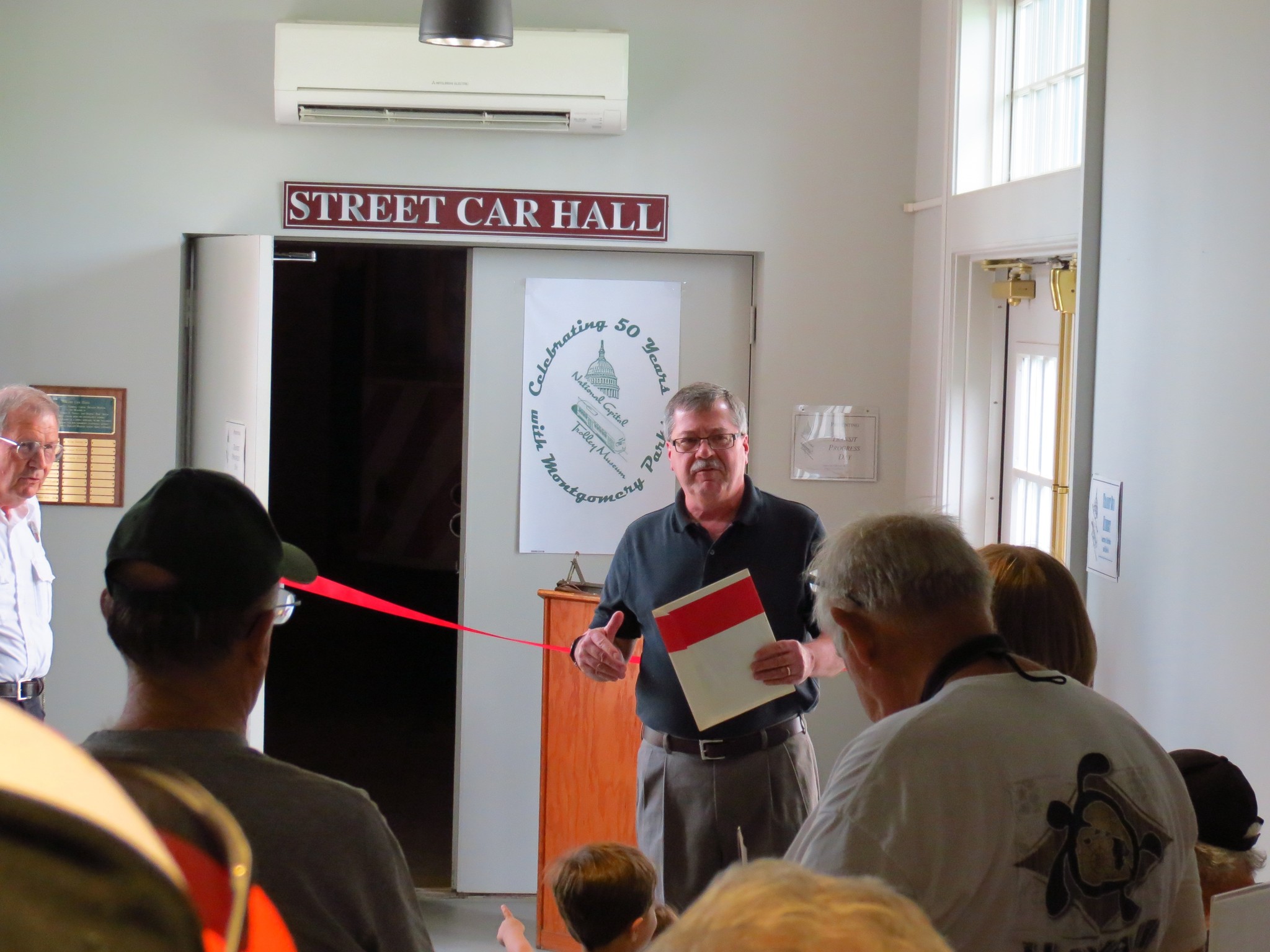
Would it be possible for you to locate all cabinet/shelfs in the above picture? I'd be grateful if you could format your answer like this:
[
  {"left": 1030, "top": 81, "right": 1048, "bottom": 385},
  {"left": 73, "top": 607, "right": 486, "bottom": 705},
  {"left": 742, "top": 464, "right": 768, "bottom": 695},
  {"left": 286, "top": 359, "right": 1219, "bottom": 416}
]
[{"left": 528, "top": 588, "right": 652, "bottom": 949}]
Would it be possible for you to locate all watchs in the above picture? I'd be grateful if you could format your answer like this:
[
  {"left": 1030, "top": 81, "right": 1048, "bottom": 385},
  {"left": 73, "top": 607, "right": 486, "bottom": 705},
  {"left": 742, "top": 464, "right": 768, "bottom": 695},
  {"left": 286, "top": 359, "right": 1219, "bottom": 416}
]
[
  {"left": 570, "top": 634, "right": 586, "bottom": 672},
  {"left": 784, "top": 503, "right": 1206, "bottom": 952}
]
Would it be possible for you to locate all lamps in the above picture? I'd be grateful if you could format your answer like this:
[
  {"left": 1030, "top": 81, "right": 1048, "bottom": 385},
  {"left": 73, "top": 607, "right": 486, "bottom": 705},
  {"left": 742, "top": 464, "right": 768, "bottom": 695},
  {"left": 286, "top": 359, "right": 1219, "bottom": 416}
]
[{"left": 414, "top": 0, "right": 515, "bottom": 51}]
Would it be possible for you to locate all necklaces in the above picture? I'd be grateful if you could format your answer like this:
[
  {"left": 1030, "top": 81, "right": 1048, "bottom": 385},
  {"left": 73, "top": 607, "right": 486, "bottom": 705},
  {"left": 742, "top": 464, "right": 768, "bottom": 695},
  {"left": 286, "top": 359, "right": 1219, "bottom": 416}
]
[{"left": 921, "top": 633, "right": 1067, "bottom": 702}]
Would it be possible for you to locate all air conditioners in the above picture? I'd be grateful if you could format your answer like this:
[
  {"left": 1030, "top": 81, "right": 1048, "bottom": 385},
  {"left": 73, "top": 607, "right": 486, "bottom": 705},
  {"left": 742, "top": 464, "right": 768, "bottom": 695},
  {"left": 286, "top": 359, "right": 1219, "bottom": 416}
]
[{"left": 270, "top": 18, "right": 631, "bottom": 144}]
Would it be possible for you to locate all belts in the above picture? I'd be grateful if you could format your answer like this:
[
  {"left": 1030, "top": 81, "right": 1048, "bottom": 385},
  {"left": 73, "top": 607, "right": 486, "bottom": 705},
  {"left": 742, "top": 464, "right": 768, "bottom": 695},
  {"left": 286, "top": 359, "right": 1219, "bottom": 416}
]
[
  {"left": 0, "top": 677, "right": 46, "bottom": 700},
  {"left": 641, "top": 716, "right": 805, "bottom": 760}
]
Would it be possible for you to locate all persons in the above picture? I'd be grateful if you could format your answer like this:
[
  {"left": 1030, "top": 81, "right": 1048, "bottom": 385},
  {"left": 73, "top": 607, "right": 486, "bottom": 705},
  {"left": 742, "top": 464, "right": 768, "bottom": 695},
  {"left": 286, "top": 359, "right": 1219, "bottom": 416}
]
[
  {"left": 1168, "top": 749, "right": 1268, "bottom": 915},
  {"left": 78, "top": 466, "right": 432, "bottom": 952},
  {"left": 650, "top": 856, "right": 957, "bottom": 952},
  {"left": 496, "top": 842, "right": 657, "bottom": 952},
  {"left": 971, "top": 544, "right": 1099, "bottom": 686},
  {"left": 573, "top": 382, "right": 846, "bottom": 921},
  {"left": 0, "top": 384, "right": 64, "bottom": 725}
]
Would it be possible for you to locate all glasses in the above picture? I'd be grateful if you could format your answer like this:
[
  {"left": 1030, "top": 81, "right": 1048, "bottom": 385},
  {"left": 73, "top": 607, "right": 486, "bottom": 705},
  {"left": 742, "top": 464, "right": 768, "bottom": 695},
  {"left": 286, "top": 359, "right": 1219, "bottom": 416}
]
[
  {"left": 0, "top": 436, "right": 65, "bottom": 462},
  {"left": 248, "top": 588, "right": 301, "bottom": 637},
  {"left": 668, "top": 433, "right": 744, "bottom": 453}
]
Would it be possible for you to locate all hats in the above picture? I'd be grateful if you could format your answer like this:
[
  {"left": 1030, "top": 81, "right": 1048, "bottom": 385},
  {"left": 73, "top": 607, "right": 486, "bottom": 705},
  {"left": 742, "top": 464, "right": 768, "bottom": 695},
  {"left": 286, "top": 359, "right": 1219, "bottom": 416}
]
[
  {"left": 106, "top": 464, "right": 317, "bottom": 614},
  {"left": 1167, "top": 749, "right": 1264, "bottom": 850}
]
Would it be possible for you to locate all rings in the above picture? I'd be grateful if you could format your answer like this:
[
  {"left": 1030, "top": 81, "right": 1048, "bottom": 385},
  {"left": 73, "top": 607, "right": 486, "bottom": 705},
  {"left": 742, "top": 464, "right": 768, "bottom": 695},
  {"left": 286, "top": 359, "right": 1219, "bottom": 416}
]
[{"left": 786, "top": 666, "right": 790, "bottom": 677}]
